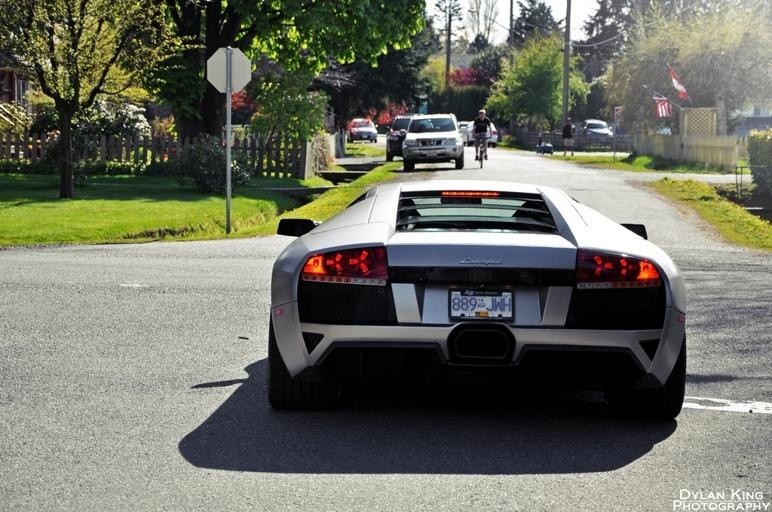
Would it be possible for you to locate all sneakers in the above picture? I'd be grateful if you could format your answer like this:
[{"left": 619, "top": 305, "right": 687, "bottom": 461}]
[
  {"left": 475, "top": 155, "right": 478, "bottom": 160},
  {"left": 484, "top": 154, "right": 488, "bottom": 160}
]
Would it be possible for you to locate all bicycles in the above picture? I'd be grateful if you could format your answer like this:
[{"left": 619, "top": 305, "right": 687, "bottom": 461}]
[{"left": 473, "top": 136, "right": 492, "bottom": 169}]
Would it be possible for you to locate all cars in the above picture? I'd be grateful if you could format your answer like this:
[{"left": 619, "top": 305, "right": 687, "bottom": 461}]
[
  {"left": 346, "top": 118, "right": 378, "bottom": 143},
  {"left": 581, "top": 119, "right": 614, "bottom": 138},
  {"left": 464, "top": 120, "right": 498, "bottom": 148}
]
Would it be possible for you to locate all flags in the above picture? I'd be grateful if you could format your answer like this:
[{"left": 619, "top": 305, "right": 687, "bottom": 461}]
[
  {"left": 666, "top": 63, "right": 691, "bottom": 101},
  {"left": 652, "top": 97, "right": 673, "bottom": 119}
]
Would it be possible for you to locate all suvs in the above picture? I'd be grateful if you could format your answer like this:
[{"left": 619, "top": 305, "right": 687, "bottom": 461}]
[
  {"left": 398, "top": 112, "right": 464, "bottom": 170},
  {"left": 386, "top": 115, "right": 414, "bottom": 162}
]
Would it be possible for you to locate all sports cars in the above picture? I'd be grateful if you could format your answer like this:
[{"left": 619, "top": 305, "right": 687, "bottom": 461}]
[{"left": 266, "top": 177, "right": 691, "bottom": 425}]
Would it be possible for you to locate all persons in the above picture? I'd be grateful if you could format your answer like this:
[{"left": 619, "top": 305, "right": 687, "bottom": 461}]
[
  {"left": 563, "top": 116, "right": 576, "bottom": 156},
  {"left": 472, "top": 109, "right": 492, "bottom": 160}
]
